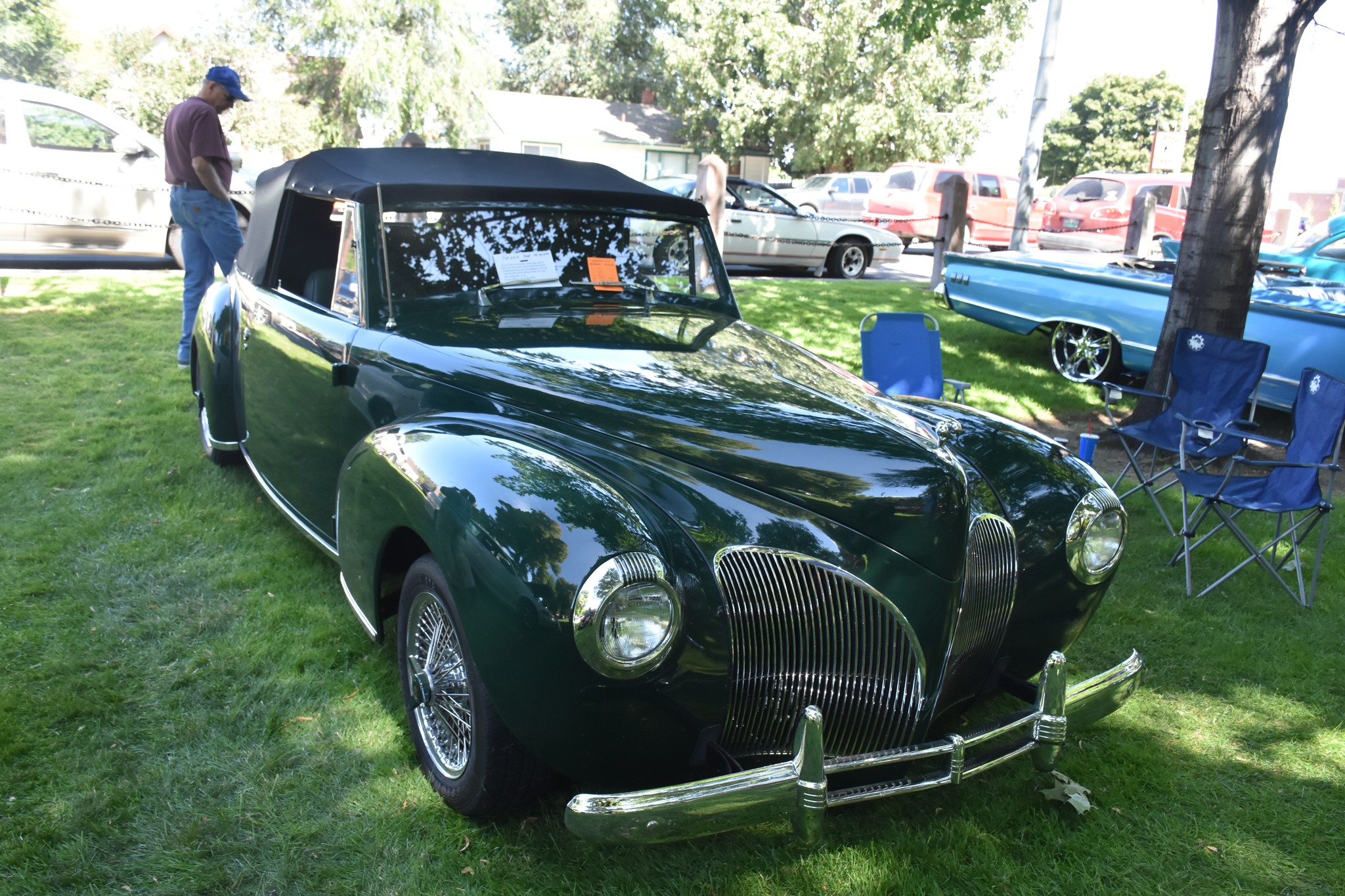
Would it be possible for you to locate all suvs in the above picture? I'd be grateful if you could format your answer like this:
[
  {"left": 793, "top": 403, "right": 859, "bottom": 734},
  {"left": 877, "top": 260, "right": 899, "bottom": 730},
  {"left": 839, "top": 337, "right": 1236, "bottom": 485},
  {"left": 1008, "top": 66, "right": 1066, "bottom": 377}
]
[
  {"left": 759, "top": 171, "right": 883, "bottom": 223},
  {"left": 1034, "top": 169, "right": 1277, "bottom": 253},
  {"left": 860, "top": 161, "right": 1049, "bottom": 252}
]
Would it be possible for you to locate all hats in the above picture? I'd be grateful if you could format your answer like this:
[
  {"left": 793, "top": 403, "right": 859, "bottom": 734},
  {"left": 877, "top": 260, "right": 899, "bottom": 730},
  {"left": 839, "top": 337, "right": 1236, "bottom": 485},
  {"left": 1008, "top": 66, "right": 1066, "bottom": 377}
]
[{"left": 205, "top": 65, "right": 250, "bottom": 102}]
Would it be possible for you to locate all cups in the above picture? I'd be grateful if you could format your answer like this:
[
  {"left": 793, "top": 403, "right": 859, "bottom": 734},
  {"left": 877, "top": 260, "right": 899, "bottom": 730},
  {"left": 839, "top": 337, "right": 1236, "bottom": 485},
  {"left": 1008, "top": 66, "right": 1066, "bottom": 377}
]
[
  {"left": 229, "top": 150, "right": 242, "bottom": 172},
  {"left": 1078, "top": 432, "right": 1100, "bottom": 466}
]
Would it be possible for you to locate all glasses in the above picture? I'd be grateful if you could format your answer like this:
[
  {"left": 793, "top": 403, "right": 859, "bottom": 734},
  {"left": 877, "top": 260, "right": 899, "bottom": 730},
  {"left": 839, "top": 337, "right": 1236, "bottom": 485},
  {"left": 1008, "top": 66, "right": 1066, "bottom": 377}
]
[{"left": 218, "top": 83, "right": 237, "bottom": 103}]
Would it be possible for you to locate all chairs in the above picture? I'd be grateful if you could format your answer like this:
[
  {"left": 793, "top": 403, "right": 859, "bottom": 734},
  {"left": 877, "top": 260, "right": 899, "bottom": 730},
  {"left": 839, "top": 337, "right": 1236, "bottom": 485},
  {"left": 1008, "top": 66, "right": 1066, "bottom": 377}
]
[
  {"left": 981, "top": 187, "right": 991, "bottom": 197},
  {"left": 303, "top": 270, "right": 426, "bottom": 308},
  {"left": 1163, "top": 367, "right": 1345, "bottom": 607},
  {"left": 860, "top": 312, "right": 971, "bottom": 404},
  {"left": 1084, "top": 327, "right": 1271, "bottom": 538}
]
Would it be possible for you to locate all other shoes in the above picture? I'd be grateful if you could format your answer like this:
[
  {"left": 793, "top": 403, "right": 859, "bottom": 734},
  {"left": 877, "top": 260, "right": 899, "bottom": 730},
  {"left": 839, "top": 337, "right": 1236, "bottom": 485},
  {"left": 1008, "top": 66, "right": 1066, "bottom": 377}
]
[{"left": 177, "top": 360, "right": 190, "bottom": 368}]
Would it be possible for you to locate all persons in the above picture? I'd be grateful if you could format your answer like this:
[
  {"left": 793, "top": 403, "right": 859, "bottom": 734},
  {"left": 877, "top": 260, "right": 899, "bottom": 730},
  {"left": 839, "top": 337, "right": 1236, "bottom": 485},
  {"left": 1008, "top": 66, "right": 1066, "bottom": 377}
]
[{"left": 164, "top": 66, "right": 254, "bottom": 368}]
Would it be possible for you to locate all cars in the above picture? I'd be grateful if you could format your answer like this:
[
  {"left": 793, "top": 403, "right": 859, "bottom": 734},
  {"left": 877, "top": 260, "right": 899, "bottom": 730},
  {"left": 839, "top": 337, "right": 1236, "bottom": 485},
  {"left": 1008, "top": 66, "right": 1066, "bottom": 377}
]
[
  {"left": 190, "top": 148, "right": 1143, "bottom": 846},
  {"left": 932, "top": 214, "right": 1345, "bottom": 412},
  {"left": 623, "top": 173, "right": 904, "bottom": 279},
  {"left": 0, "top": 79, "right": 256, "bottom": 270}
]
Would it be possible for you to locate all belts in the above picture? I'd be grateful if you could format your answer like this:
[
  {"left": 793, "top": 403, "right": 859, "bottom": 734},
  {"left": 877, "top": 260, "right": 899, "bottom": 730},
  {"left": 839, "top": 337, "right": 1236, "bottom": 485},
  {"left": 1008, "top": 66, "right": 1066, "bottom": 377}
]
[{"left": 173, "top": 184, "right": 206, "bottom": 190}]
